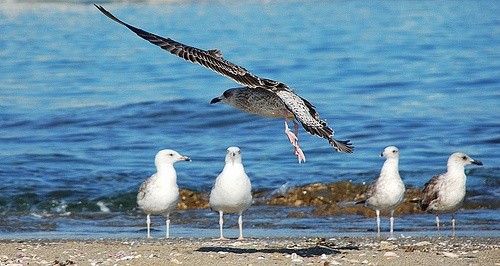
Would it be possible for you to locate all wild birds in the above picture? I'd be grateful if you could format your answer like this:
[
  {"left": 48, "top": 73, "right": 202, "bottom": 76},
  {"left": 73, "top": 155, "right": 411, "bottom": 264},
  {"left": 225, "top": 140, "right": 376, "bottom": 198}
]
[
  {"left": 343, "top": 143, "right": 408, "bottom": 241},
  {"left": 409, "top": 152, "right": 484, "bottom": 242},
  {"left": 206, "top": 145, "right": 254, "bottom": 242},
  {"left": 91, "top": 2, "right": 355, "bottom": 165},
  {"left": 134, "top": 147, "right": 193, "bottom": 240}
]
